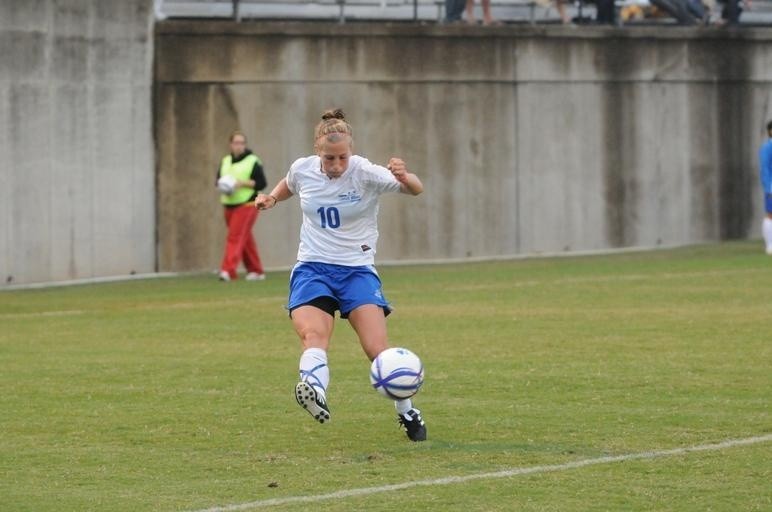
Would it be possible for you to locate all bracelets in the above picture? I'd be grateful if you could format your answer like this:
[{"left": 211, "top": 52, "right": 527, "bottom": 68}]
[{"left": 270, "top": 196, "right": 277, "bottom": 205}]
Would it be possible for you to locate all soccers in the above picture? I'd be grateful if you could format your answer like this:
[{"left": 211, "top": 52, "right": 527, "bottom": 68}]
[
  {"left": 370, "top": 347, "right": 426, "bottom": 400},
  {"left": 216, "top": 177, "right": 237, "bottom": 195}
]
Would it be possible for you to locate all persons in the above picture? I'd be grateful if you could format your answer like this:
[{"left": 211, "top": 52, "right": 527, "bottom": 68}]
[
  {"left": 534, "top": 1, "right": 753, "bottom": 27},
  {"left": 759, "top": 120, "right": 772, "bottom": 255},
  {"left": 444, "top": 0, "right": 492, "bottom": 27},
  {"left": 254, "top": 108, "right": 427, "bottom": 443},
  {"left": 215, "top": 131, "right": 267, "bottom": 282}
]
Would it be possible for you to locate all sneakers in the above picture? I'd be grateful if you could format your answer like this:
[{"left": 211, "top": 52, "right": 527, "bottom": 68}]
[
  {"left": 295, "top": 380, "right": 331, "bottom": 424},
  {"left": 245, "top": 271, "right": 265, "bottom": 281},
  {"left": 219, "top": 270, "right": 232, "bottom": 282},
  {"left": 398, "top": 408, "right": 427, "bottom": 441}
]
